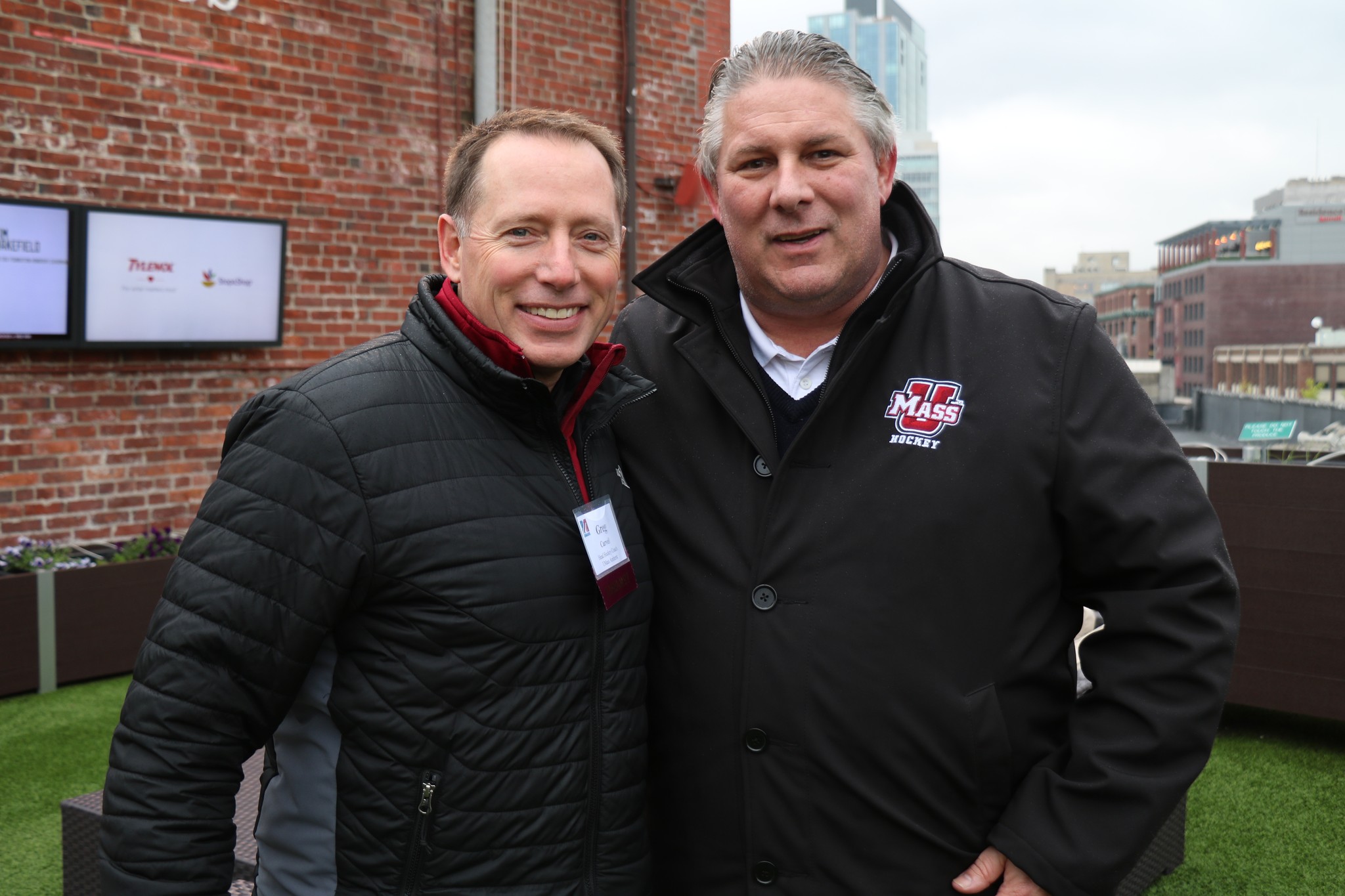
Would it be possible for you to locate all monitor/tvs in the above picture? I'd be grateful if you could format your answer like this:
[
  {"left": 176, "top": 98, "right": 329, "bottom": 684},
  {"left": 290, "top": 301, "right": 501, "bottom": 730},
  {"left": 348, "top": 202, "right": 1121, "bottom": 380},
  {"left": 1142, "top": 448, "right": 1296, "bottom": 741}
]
[{"left": 0, "top": 196, "right": 288, "bottom": 351}]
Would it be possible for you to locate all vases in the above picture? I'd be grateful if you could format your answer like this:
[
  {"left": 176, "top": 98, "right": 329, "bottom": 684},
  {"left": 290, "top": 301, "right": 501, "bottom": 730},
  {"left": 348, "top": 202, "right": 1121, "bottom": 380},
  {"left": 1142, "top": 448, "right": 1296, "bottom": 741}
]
[{"left": 0, "top": 541, "right": 176, "bottom": 696}]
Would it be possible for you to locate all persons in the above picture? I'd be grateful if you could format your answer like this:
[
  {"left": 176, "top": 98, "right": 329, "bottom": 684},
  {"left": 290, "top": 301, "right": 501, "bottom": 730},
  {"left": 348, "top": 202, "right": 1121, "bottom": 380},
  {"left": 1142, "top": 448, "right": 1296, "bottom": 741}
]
[
  {"left": 611, "top": 28, "right": 1244, "bottom": 895},
  {"left": 95, "top": 104, "right": 656, "bottom": 895}
]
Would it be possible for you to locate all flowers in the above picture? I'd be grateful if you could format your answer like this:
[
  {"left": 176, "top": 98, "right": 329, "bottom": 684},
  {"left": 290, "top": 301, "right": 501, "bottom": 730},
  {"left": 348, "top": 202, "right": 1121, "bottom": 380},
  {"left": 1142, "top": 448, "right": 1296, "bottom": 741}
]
[{"left": 0, "top": 526, "right": 181, "bottom": 570}]
